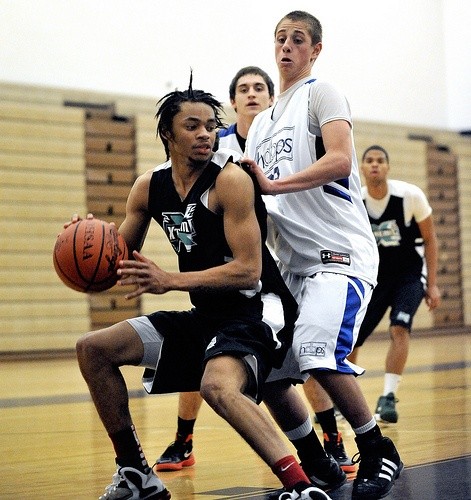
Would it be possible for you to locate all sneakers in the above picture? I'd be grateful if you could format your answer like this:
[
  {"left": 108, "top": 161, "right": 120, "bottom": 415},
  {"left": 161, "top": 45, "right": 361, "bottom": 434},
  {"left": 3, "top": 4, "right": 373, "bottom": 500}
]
[
  {"left": 155, "top": 441, "right": 195, "bottom": 470},
  {"left": 99, "top": 456, "right": 171, "bottom": 500},
  {"left": 347, "top": 436, "right": 405, "bottom": 500},
  {"left": 297, "top": 454, "right": 346, "bottom": 493},
  {"left": 279, "top": 482, "right": 332, "bottom": 500},
  {"left": 374, "top": 391, "right": 400, "bottom": 423},
  {"left": 325, "top": 450, "right": 354, "bottom": 473}
]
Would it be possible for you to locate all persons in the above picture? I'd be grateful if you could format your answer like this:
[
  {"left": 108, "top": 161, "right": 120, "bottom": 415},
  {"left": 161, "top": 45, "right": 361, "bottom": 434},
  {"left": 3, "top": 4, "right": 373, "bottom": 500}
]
[
  {"left": 241, "top": 9, "right": 404, "bottom": 500},
  {"left": 140, "top": 65, "right": 357, "bottom": 472},
  {"left": 56, "top": 88, "right": 332, "bottom": 500},
  {"left": 345, "top": 144, "right": 440, "bottom": 424}
]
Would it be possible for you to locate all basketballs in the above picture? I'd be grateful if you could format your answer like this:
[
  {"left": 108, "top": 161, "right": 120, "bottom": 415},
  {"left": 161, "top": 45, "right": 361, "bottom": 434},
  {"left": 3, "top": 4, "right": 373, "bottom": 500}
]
[{"left": 52, "top": 218, "right": 129, "bottom": 294}]
